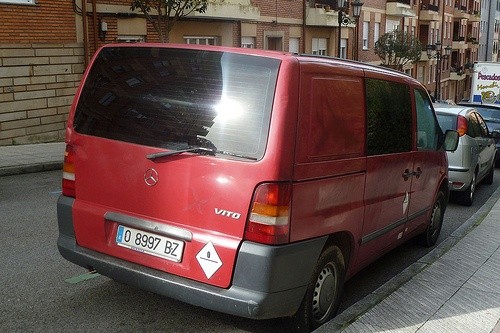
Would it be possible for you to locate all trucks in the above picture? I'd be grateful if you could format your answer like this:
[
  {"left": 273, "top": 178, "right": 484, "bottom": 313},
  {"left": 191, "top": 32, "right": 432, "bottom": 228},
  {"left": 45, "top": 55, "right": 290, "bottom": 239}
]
[{"left": 469, "top": 61, "right": 500, "bottom": 105}]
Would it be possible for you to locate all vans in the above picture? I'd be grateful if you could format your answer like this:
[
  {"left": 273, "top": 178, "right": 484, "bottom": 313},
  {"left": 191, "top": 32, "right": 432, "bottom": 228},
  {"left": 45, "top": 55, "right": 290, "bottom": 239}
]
[{"left": 56, "top": 43, "right": 460, "bottom": 333}]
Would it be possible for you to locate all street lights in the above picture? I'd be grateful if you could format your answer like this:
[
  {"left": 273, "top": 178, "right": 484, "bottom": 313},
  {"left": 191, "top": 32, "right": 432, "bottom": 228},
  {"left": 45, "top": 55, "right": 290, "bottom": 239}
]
[
  {"left": 434, "top": 40, "right": 441, "bottom": 101},
  {"left": 337, "top": 0, "right": 364, "bottom": 58}
]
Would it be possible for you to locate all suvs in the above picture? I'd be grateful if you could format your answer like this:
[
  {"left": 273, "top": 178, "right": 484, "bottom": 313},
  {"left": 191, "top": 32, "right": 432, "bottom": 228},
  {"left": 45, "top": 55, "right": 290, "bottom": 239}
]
[
  {"left": 457, "top": 101, "right": 500, "bottom": 169},
  {"left": 432, "top": 102, "right": 496, "bottom": 206}
]
